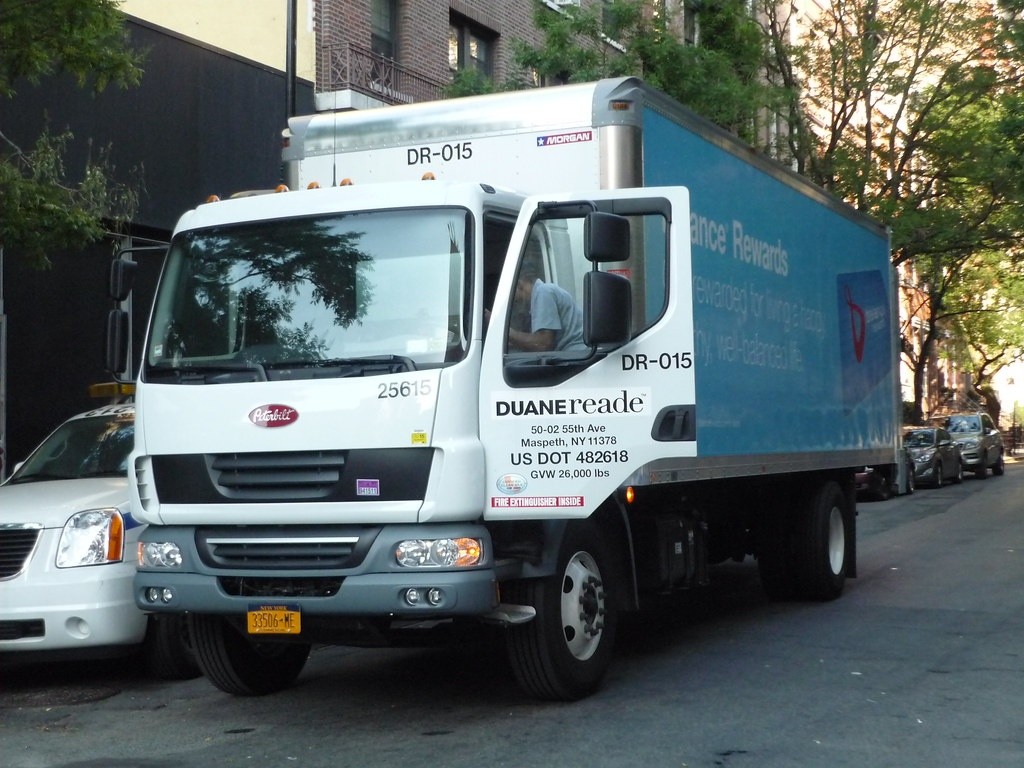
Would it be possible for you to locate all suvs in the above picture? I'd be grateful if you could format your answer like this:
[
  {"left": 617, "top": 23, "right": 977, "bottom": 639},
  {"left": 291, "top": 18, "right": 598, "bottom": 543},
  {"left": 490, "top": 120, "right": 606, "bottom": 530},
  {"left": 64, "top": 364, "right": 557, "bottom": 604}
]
[{"left": 925, "top": 411, "right": 1005, "bottom": 479}]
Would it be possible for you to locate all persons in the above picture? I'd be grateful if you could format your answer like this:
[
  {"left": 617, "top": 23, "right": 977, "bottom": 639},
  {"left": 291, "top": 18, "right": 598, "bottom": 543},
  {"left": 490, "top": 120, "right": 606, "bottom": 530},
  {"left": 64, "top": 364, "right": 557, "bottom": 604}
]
[{"left": 481, "top": 262, "right": 588, "bottom": 352}]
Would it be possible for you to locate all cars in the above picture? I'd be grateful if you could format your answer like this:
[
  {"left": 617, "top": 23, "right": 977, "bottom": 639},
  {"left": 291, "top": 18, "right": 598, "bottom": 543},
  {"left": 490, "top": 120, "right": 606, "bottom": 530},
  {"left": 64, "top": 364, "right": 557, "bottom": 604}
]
[
  {"left": 1, "top": 383, "right": 202, "bottom": 680},
  {"left": 855, "top": 453, "right": 915, "bottom": 496},
  {"left": 904, "top": 428, "right": 963, "bottom": 485}
]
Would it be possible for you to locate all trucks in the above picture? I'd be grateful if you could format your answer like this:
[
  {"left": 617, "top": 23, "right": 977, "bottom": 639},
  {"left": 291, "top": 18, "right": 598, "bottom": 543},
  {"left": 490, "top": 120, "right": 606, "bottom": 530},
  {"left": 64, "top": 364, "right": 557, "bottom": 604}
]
[{"left": 129, "top": 77, "right": 905, "bottom": 698}]
